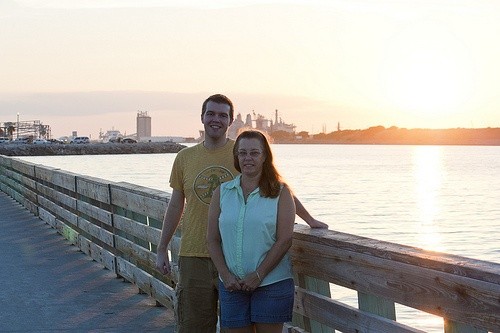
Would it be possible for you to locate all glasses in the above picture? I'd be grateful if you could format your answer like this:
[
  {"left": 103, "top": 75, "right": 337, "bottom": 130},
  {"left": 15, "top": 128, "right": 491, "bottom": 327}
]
[{"left": 235, "top": 151, "right": 260, "bottom": 157}]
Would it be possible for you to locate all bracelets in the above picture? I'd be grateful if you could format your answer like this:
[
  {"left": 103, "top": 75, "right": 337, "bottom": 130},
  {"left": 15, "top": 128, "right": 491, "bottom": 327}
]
[{"left": 256, "top": 271, "right": 262, "bottom": 283}]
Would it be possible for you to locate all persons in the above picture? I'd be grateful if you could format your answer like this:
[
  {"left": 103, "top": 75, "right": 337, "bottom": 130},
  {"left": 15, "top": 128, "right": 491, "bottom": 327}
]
[
  {"left": 206, "top": 129, "right": 296, "bottom": 333},
  {"left": 156, "top": 93, "right": 329, "bottom": 333}
]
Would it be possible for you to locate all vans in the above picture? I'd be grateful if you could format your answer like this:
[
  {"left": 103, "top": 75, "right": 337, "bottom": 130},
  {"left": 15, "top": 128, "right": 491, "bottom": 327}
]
[{"left": 70, "top": 136, "right": 90, "bottom": 144}]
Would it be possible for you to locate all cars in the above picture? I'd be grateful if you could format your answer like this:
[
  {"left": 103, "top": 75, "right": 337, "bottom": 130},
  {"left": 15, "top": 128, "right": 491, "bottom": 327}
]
[
  {"left": 0, "top": 137, "right": 64, "bottom": 145},
  {"left": 122, "top": 138, "right": 137, "bottom": 144}
]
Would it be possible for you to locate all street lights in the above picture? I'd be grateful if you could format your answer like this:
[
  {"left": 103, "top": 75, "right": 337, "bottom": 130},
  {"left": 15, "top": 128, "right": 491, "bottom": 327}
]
[{"left": 17, "top": 112, "right": 20, "bottom": 139}]
